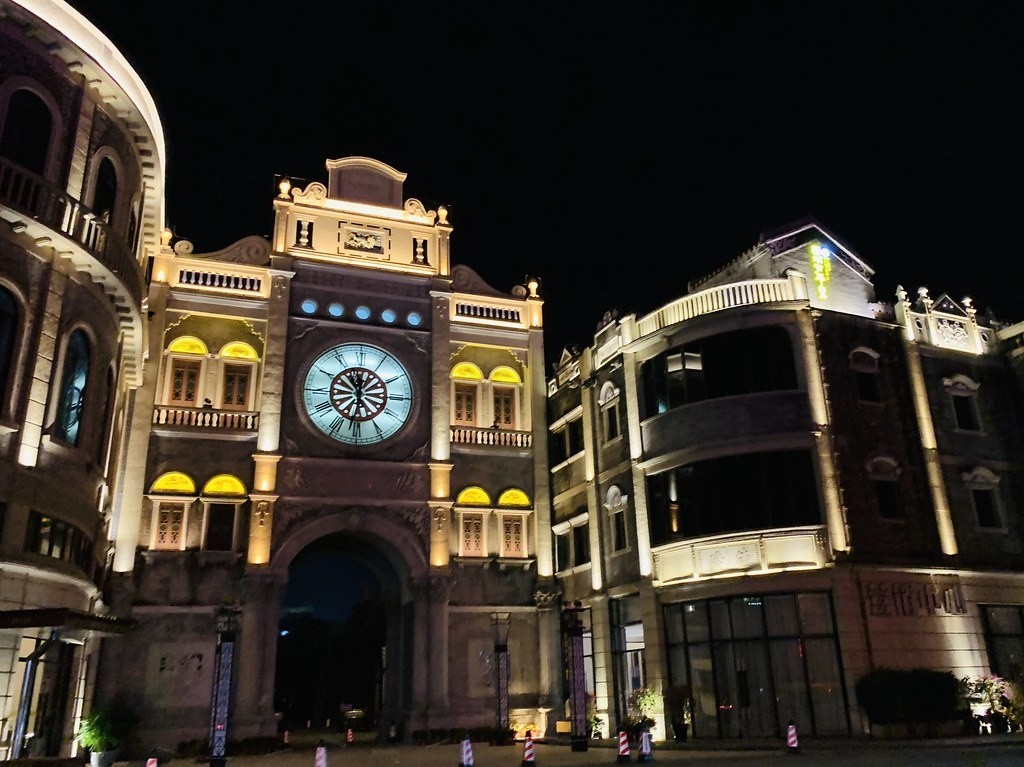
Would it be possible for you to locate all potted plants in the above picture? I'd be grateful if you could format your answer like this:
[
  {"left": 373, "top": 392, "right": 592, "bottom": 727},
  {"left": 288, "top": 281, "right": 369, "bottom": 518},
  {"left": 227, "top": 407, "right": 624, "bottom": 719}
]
[
  {"left": 855, "top": 668, "right": 970, "bottom": 741},
  {"left": 566, "top": 694, "right": 605, "bottom": 739},
  {"left": 65, "top": 708, "right": 122, "bottom": 767}
]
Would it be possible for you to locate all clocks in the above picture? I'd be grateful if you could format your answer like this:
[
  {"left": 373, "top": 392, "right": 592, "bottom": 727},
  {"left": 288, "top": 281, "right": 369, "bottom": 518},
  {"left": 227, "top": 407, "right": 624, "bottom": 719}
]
[{"left": 295, "top": 336, "right": 421, "bottom": 454}]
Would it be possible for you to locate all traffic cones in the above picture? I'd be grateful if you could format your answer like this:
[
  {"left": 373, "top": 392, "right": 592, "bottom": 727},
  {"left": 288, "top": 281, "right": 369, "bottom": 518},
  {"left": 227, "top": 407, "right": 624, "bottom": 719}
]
[
  {"left": 281, "top": 728, "right": 291, "bottom": 750},
  {"left": 344, "top": 725, "right": 353, "bottom": 743},
  {"left": 388, "top": 721, "right": 398, "bottom": 743},
  {"left": 146, "top": 751, "right": 158, "bottom": 767},
  {"left": 637, "top": 724, "right": 654, "bottom": 761},
  {"left": 783, "top": 722, "right": 800, "bottom": 755},
  {"left": 615, "top": 728, "right": 632, "bottom": 763},
  {"left": 313, "top": 738, "right": 328, "bottom": 767},
  {"left": 458, "top": 732, "right": 475, "bottom": 767},
  {"left": 326, "top": 718, "right": 331, "bottom": 730},
  {"left": 306, "top": 719, "right": 312, "bottom": 728},
  {"left": 520, "top": 728, "right": 538, "bottom": 767}
]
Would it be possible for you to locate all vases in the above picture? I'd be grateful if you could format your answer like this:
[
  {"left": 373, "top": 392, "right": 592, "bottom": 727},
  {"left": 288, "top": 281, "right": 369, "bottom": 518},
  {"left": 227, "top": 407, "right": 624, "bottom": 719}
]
[
  {"left": 614, "top": 718, "right": 656, "bottom": 746},
  {"left": 982, "top": 711, "right": 1007, "bottom": 735}
]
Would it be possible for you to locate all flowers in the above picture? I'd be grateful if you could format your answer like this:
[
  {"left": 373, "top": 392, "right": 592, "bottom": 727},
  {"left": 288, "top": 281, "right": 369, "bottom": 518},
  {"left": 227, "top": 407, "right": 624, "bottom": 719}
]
[
  {"left": 973, "top": 673, "right": 1011, "bottom": 713},
  {"left": 625, "top": 686, "right": 656, "bottom": 720}
]
